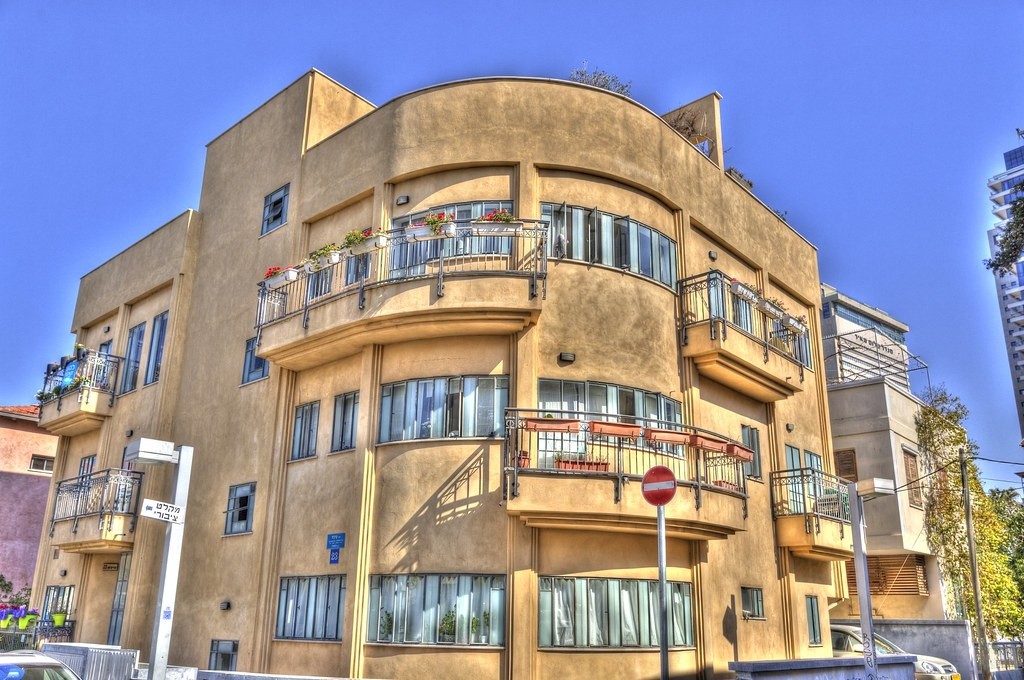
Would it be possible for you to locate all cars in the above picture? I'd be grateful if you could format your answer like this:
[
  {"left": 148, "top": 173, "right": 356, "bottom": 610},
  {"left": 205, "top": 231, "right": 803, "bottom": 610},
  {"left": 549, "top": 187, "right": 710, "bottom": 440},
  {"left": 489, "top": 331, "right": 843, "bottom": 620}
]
[
  {"left": 0, "top": 649, "right": 82, "bottom": 680},
  {"left": 830, "top": 625, "right": 961, "bottom": 680}
]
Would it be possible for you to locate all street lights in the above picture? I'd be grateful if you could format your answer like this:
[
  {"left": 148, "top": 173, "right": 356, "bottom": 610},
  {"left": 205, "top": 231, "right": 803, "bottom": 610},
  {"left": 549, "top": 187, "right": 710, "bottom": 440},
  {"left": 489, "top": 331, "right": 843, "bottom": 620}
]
[
  {"left": 124, "top": 437, "right": 194, "bottom": 680},
  {"left": 848, "top": 478, "right": 895, "bottom": 680}
]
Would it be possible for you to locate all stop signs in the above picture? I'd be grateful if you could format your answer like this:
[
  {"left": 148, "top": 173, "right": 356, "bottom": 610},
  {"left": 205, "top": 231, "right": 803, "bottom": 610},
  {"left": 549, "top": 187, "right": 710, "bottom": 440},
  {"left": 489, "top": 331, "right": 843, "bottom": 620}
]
[{"left": 641, "top": 466, "right": 677, "bottom": 506}]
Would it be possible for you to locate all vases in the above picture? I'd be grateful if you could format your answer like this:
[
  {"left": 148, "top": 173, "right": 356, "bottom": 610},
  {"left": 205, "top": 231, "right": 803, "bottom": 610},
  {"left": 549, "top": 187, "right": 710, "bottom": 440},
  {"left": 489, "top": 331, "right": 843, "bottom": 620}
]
[
  {"left": 265, "top": 268, "right": 299, "bottom": 290},
  {"left": 512, "top": 456, "right": 530, "bottom": 469},
  {"left": 405, "top": 222, "right": 456, "bottom": 243},
  {"left": 782, "top": 315, "right": 805, "bottom": 335},
  {"left": 731, "top": 282, "right": 759, "bottom": 304},
  {"left": 588, "top": 420, "right": 756, "bottom": 462},
  {"left": 19, "top": 615, "right": 37, "bottom": 629},
  {"left": 712, "top": 480, "right": 737, "bottom": 490},
  {"left": 756, "top": 299, "right": 784, "bottom": 320},
  {"left": 0, "top": 615, "right": 14, "bottom": 629},
  {"left": 524, "top": 416, "right": 581, "bottom": 433},
  {"left": 346, "top": 235, "right": 391, "bottom": 257},
  {"left": 470, "top": 220, "right": 523, "bottom": 236}
]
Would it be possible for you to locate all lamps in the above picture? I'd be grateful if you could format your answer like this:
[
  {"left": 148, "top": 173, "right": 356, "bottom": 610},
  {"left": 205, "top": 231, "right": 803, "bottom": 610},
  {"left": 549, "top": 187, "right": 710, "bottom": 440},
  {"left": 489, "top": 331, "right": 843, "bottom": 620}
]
[
  {"left": 560, "top": 353, "right": 575, "bottom": 361},
  {"left": 103, "top": 326, "right": 110, "bottom": 333},
  {"left": 709, "top": 250, "right": 718, "bottom": 261},
  {"left": 125, "top": 430, "right": 134, "bottom": 437},
  {"left": 60, "top": 570, "right": 67, "bottom": 576},
  {"left": 396, "top": 195, "right": 410, "bottom": 205},
  {"left": 221, "top": 601, "right": 231, "bottom": 609},
  {"left": 787, "top": 423, "right": 795, "bottom": 430}
]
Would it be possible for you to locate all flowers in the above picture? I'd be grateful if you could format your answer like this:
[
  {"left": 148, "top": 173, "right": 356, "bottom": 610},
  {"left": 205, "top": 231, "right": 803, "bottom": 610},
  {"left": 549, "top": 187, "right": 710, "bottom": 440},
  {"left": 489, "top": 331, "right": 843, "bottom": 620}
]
[
  {"left": 786, "top": 311, "right": 809, "bottom": 332},
  {"left": 263, "top": 265, "right": 294, "bottom": 280},
  {"left": 408, "top": 211, "right": 454, "bottom": 235},
  {"left": 730, "top": 277, "right": 762, "bottom": 306},
  {"left": 33, "top": 373, "right": 93, "bottom": 402},
  {"left": 345, "top": 227, "right": 390, "bottom": 248},
  {"left": 766, "top": 296, "right": 790, "bottom": 320},
  {"left": 0, "top": 603, "right": 41, "bottom": 619},
  {"left": 476, "top": 208, "right": 523, "bottom": 222}
]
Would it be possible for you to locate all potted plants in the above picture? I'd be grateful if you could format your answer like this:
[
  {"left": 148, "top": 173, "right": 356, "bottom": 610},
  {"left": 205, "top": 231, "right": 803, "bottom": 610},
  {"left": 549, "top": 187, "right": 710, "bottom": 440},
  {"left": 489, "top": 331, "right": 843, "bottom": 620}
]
[
  {"left": 554, "top": 452, "right": 610, "bottom": 472},
  {"left": 299, "top": 245, "right": 342, "bottom": 274},
  {"left": 60, "top": 355, "right": 71, "bottom": 368},
  {"left": 378, "top": 605, "right": 489, "bottom": 645},
  {"left": 74, "top": 343, "right": 86, "bottom": 361},
  {"left": 47, "top": 594, "right": 77, "bottom": 628},
  {"left": 46, "top": 362, "right": 59, "bottom": 376}
]
